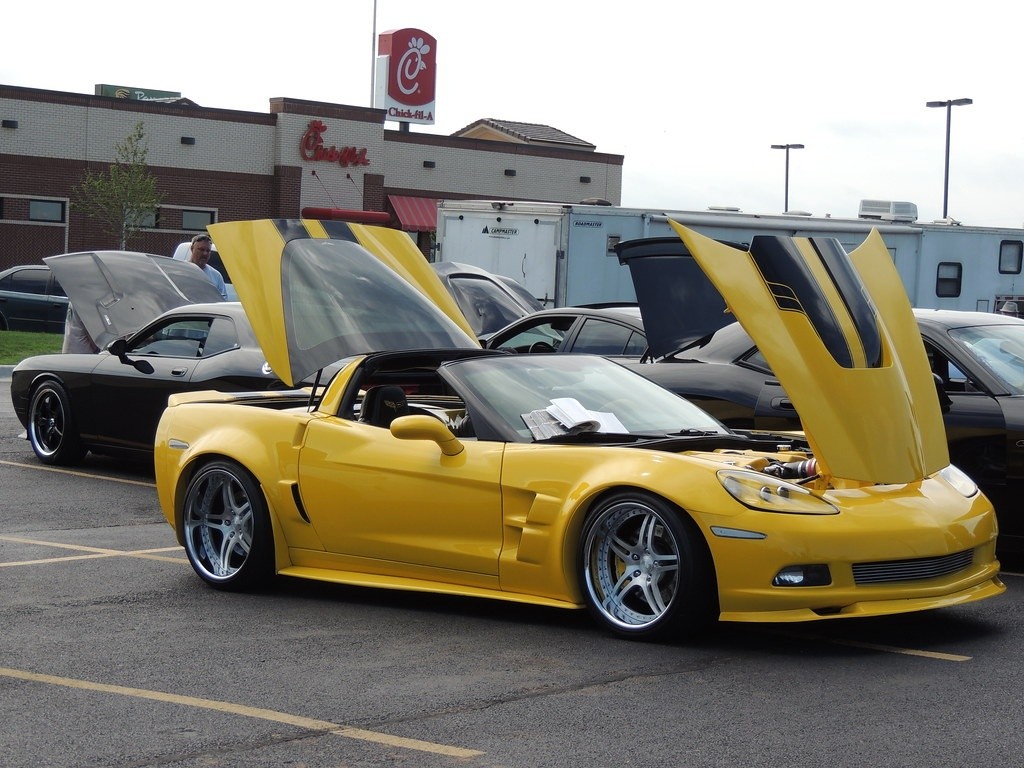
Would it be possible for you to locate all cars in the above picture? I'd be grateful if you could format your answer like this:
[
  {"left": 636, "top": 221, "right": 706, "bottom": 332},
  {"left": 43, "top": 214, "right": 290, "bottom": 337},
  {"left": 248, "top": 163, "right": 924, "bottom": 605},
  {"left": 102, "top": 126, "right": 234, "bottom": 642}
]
[
  {"left": 0, "top": 264, "right": 70, "bottom": 329},
  {"left": 590, "top": 236, "right": 1024, "bottom": 520},
  {"left": 427, "top": 261, "right": 649, "bottom": 361}
]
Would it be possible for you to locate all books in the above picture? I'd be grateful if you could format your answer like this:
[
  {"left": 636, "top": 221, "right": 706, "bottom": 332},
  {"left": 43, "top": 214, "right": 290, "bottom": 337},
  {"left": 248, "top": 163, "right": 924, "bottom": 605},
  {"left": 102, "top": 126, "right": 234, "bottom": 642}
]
[{"left": 521, "top": 397, "right": 629, "bottom": 440}]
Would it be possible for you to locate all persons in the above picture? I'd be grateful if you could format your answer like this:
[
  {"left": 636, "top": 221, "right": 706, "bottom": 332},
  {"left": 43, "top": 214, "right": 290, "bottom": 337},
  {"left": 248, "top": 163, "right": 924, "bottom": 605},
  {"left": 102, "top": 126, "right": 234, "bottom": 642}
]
[
  {"left": 185, "top": 234, "right": 229, "bottom": 302},
  {"left": 998, "top": 301, "right": 1019, "bottom": 317},
  {"left": 62, "top": 301, "right": 98, "bottom": 355}
]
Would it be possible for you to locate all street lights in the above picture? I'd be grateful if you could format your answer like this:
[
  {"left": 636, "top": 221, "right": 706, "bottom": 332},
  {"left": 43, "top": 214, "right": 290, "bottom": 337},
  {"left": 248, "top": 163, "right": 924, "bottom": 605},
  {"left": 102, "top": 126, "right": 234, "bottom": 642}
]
[
  {"left": 772, "top": 144, "right": 806, "bottom": 216},
  {"left": 927, "top": 98, "right": 973, "bottom": 222}
]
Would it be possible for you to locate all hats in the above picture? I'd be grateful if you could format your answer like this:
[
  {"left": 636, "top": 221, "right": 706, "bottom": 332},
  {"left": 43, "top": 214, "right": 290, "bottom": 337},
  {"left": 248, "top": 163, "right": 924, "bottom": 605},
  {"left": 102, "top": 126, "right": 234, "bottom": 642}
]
[{"left": 1000, "top": 302, "right": 1019, "bottom": 312}]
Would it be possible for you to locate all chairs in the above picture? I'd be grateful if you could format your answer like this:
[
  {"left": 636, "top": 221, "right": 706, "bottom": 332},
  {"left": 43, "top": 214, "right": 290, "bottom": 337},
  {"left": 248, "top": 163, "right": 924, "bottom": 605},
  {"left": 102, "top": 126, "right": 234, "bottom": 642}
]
[{"left": 358, "top": 385, "right": 513, "bottom": 438}]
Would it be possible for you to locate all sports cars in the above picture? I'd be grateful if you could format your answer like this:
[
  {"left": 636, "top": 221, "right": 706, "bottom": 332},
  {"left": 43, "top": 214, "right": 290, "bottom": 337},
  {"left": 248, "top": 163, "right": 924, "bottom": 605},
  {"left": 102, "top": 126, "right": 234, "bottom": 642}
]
[
  {"left": 152, "top": 219, "right": 1008, "bottom": 639},
  {"left": 10, "top": 250, "right": 474, "bottom": 466},
  {"left": 428, "top": 238, "right": 954, "bottom": 465}
]
